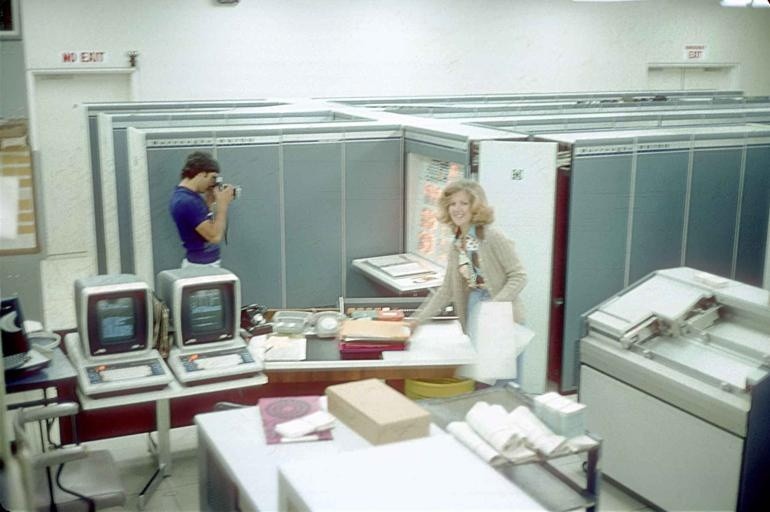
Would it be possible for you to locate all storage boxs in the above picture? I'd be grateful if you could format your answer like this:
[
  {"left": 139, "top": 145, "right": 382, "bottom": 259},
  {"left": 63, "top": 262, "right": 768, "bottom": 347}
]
[{"left": 324, "top": 377, "right": 430, "bottom": 446}]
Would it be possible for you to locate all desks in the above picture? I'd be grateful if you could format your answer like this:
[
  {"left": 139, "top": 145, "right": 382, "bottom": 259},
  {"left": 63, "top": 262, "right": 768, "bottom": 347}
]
[{"left": 4, "top": 331, "right": 78, "bottom": 446}]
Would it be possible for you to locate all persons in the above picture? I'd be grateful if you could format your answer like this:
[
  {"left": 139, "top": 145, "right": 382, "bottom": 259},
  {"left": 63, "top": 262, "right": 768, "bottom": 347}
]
[
  {"left": 404, "top": 179, "right": 527, "bottom": 388},
  {"left": 169, "top": 153, "right": 235, "bottom": 269}
]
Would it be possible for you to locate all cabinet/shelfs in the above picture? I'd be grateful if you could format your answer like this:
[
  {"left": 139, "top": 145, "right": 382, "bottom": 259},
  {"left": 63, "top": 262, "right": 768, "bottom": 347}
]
[{"left": 412, "top": 383, "right": 603, "bottom": 512}]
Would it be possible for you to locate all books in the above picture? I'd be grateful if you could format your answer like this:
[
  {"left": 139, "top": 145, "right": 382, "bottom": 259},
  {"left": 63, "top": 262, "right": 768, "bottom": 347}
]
[{"left": 339, "top": 319, "right": 411, "bottom": 360}]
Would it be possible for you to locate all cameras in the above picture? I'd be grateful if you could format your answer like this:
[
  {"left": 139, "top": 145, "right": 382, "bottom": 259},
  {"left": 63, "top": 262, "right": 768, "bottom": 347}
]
[{"left": 213, "top": 176, "right": 241, "bottom": 201}]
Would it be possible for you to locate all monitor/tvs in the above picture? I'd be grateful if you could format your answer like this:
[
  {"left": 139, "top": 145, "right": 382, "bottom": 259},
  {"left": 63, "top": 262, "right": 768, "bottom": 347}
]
[
  {"left": 154, "top": 266, "right": 241, "bottom": 351},
  {"left": 73, "top": 274, "right": 154, "bottom": 361}
]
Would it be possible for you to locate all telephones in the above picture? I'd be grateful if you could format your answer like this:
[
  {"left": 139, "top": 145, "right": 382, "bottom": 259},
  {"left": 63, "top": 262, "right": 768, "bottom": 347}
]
[{"left": 309, "top": 311, "right": 346, "bottom": 338}]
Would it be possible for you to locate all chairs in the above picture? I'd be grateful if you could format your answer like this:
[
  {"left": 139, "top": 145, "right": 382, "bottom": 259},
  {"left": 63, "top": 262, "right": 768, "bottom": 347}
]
[{"left": 10, "top": 402, "right": 126, "bottom": 512}]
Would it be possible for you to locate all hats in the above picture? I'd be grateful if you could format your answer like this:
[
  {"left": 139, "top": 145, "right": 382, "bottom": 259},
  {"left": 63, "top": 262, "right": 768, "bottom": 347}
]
[{"left": 181, "top": 152, "right": 222, "bottom": 173}]
